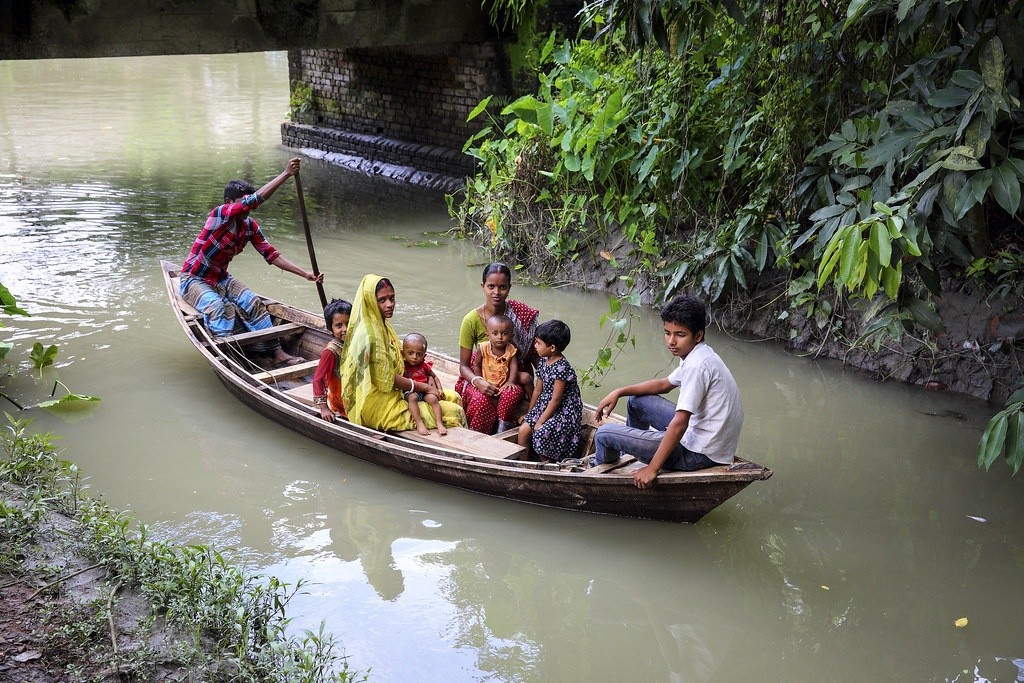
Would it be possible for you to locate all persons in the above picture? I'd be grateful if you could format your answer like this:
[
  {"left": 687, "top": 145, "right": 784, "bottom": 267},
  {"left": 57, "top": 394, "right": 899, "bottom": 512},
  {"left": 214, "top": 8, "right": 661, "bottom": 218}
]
[
  {"left": 454, "top": 261, "right": 541, "bottom": 436},
  {"left": 178, "top": 156, "right": 325, "bottom": 372},
  {"left": 473, "top": 314, "right": 538, "bottom": 403},
  {"left": 590, "top": 295, "right": 746, "bottom": 490},
  {"left": 313, "top": 297, "right": 353, "bottom": 423},
  {"left": 399, "top": 332, "right": 448, "bottom": 436},
  {"left": 338, "top": 274, "right": 471, "bottom": 432},
  {"left": 518, "top": 318, "right": 584, "bottom": 462}
]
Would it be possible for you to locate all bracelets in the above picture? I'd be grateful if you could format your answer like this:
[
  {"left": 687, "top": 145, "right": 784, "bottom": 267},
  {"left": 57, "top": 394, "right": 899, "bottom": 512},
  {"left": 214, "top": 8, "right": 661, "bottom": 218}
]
[
  {"left": 409, "top": 379, "right": 415, "bottom": 392},
  {"left": 470, "top": 376, "right": 484, "bottom": 387}
]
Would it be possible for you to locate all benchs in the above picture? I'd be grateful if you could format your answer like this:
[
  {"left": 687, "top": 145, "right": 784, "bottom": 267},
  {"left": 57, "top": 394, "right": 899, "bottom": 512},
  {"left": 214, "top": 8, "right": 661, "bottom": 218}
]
[
  {"left": 201, "top": 322, "right": 304, "bottom": 351},
  {"left": 583, "top": 454, "right": 648, "bottom": 476},
  {"left": 172, "top": 276, "right": 267, "bottom": 327},
  {"left": 251, "top": 358, "right": 320, "bottom": 384},
  {"left": 282, "top": 383, "right": 527, "bottom": 460},
  {"left": 491, "top": 425, "right": 522, "bottom": 444}
]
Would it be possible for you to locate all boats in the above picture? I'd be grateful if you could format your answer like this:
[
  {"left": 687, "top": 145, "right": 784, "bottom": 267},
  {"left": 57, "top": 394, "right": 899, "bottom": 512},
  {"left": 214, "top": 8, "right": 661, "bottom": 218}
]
[{"left": 158, "top": 259, "right": 774, "bottom": 524}]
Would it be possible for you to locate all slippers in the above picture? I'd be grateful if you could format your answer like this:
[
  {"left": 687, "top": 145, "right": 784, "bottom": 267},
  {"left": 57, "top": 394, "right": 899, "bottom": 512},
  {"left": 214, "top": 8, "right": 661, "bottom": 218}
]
[{"left": 272, "top": 356, "right": 306, "bottom": 367}]
